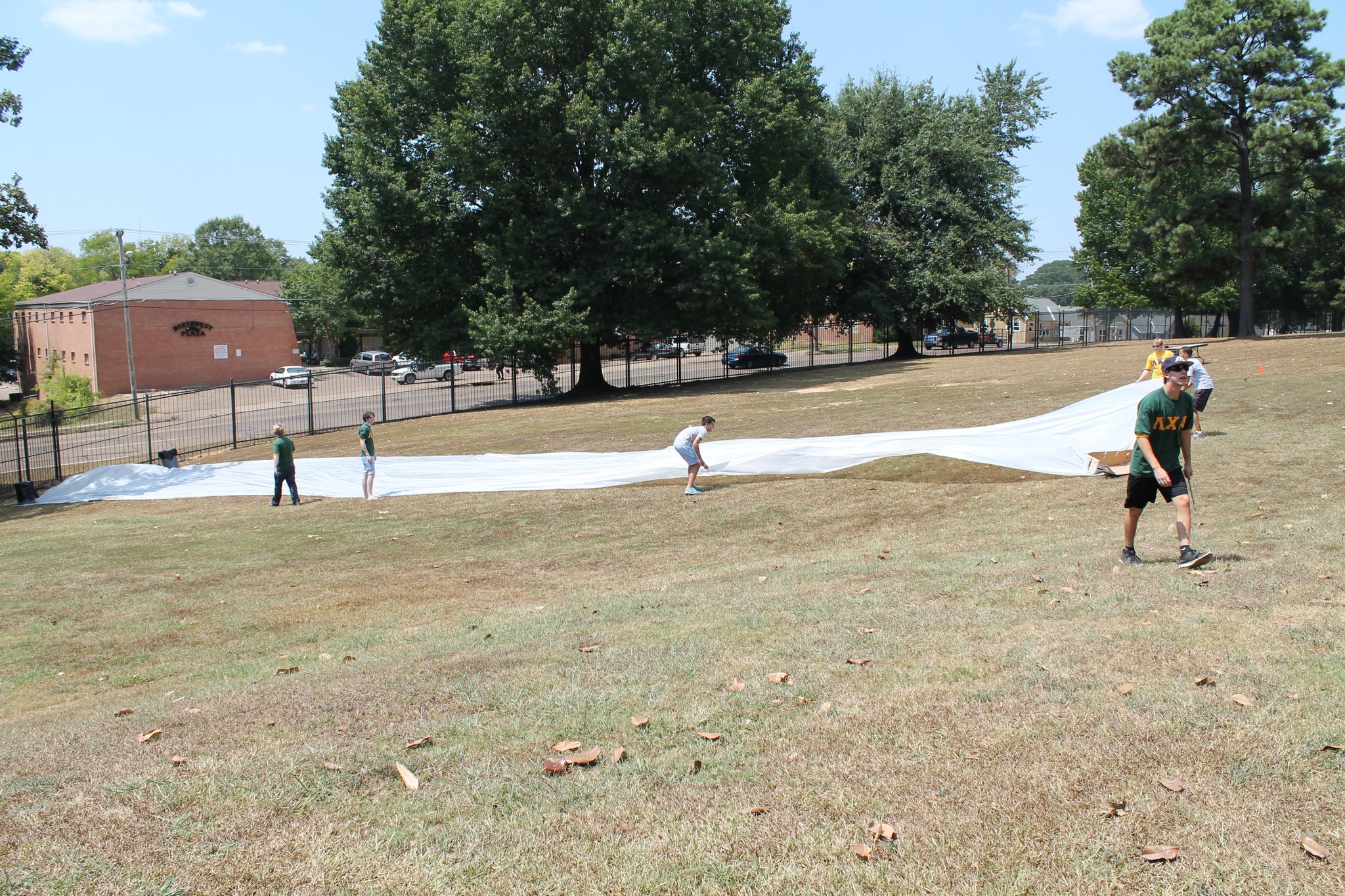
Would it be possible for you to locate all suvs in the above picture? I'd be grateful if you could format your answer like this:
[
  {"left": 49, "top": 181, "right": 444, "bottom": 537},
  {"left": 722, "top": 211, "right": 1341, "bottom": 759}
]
[
  {"left": 350, "top": 351, "right": 394, "bottom": 376},
  {"left": 659, "top": 334, "right": 706, "bottom": 358}
]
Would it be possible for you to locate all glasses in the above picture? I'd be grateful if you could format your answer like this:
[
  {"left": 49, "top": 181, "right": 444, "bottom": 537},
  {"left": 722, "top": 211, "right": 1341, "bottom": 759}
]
[
  {"left": 1152, "top": 343, "right": 1162, "bottom": 348},
  {"left": 1167, "top": 364, "right": 1189, "bottom": 372}
]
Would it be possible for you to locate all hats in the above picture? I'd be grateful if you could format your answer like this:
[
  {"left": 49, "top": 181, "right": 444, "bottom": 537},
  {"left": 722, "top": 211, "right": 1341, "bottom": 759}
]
[{"left": 1161, "top": 355, "right": 1194, "bottom": 374}]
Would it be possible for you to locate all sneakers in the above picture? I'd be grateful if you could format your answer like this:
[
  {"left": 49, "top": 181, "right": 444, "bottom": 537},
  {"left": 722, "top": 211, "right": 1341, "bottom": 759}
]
[
  {"left": 684, "top": 486, "right": 702, "bottom": 494},
  {"left": 1190, "top": 431, "right": 1193, "bottom": 436},
  {"left": 692, "top": 484, "right": 699, "bottom": 489},
  {"left": 1191, "top": 431, "right": 1205, "bottom": 438},
  {"left": 1178, "top": 549, "right": 1213, "bottom": 569},
  {"left": 1119, "top": 548, "right": 1144, "bottom": 566}
]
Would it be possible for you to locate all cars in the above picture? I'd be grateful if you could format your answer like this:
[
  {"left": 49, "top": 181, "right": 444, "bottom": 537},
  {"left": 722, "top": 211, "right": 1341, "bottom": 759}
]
[
  {"left": 392, "top": 350, "right": 422, "bottom": 370},
  {"left": 721, "top": 346, "right": 787, "bottom": 369},
  {"left": 629, "top": 343, "right": 679, "bottom": 361},
  {"left": 442, "top": 349, "right": 483, "bottom": 371},
  {"left": 269, "top": 366, "right": 315, "bottom": 389}
]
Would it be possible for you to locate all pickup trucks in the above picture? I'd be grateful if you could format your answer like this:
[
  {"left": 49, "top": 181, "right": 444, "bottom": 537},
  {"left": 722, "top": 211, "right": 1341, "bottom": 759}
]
[
  {"left": 924, "top": 326, "right": 979, "bottom": 350},
  {"left": 391, "top": 357, "right": 464, "bottom": 385}
]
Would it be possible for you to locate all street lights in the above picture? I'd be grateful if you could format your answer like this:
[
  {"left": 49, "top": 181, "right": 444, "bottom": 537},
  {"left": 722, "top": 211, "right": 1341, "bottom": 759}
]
[{"left": 119, "top": 250, "right": 140, "bottom": 422}]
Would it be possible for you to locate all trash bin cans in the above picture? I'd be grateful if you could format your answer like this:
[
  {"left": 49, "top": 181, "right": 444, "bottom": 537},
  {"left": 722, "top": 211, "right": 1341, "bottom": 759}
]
[
  {"left": 14, "top": 481, "right": 34, "bottom": 505},
  {"left": 158, "top": 448, "right": 179, "bottom": 469}
]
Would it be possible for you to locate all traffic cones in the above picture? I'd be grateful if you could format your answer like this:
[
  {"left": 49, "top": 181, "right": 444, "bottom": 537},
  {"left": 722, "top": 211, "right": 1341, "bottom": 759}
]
[{"left": 1258, "top": 365, "right": 1264, "bottom": 374}]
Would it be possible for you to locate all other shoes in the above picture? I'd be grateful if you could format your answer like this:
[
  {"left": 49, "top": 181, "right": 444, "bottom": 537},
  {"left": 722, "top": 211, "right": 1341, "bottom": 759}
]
[
  {"left": 267, "top": 502, "right": 274, "bottom": 507},
  {"left": 362, "top": 495, "right": 378, "bottom": 500},
  {"left": 293, "top": 501, "right": 301, "bottom": 506}
]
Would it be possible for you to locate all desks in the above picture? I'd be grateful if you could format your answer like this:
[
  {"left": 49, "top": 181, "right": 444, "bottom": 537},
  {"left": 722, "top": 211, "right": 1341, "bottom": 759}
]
[{"left": 1163, "top": 343, "right": 1208, "bottom": 364}]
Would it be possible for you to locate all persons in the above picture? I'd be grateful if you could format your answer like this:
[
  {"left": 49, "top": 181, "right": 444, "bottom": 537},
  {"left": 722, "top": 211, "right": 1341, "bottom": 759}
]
[
  {"left": 358, "top": 411, "right": 378, "bottom": 500},
  {"left": 267, "top": 423, "right": 301, "bottom": 507},
  {"left": 673, "top": 416, "right": 716, "bottom": 494},
  {"left": 1134, "top": 338, "right": 1174, "bottom": 384},
  {"left": 1179, "top": 347, "right": 1214, "bottom": 438},
  {"left": 1118, "top": 355, "right": 1213, "bottom": 569}
]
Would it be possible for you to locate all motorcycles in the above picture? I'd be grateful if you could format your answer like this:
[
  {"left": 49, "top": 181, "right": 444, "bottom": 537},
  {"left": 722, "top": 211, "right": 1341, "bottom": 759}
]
[{"left": 978, "top": 330, "right": 1003, "bottom": 348}]
